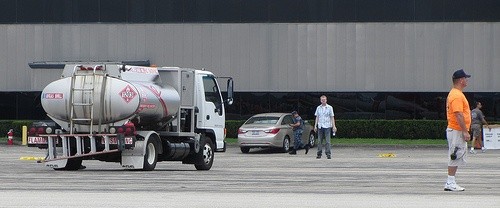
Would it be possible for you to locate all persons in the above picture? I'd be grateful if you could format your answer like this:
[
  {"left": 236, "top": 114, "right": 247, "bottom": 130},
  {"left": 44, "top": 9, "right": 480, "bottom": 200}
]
[
  {"left": 288, "top": 111, "right": 310, "bottom": 155},
  {"left": 444, "top": 69, "right": 472, "bottom": 191},
  {"left": 470, "top": 100, "right": 492, "bottom": 154},
  {"left": 314, "top": 95, "right": 337, "bottom": 159}
]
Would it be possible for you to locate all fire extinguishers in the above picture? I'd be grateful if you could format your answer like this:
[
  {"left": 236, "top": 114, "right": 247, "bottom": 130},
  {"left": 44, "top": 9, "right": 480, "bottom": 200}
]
[{"left": 6, "top": 129, "right": 14, "bottom": 144}]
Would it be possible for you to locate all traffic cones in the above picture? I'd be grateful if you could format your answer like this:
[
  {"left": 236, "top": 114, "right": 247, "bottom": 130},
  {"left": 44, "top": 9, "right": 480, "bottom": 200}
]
[{"left": 8, "top": 132, "right": 13, "bottom": 144}]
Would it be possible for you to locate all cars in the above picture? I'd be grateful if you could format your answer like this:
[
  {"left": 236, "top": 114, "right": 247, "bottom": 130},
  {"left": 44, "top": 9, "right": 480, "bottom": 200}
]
[{"left": 237, "top": 113, "right": 315, "bottom": 153}]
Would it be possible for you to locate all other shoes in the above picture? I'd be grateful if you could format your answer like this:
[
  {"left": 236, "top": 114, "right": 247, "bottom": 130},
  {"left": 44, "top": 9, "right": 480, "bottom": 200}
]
[
  {"left": 289, "top": 150, "right": 296, "bottom": 154},
  {"left": 470, "top": 150, "right": 477, "bottom": 154},
  {"left": 316, "top": 154, "right": 321, "bottom": 158},
  {"left": 305, "top": 147, "right": 310, "bottom": 154},
  {"left": 327, "top": 153, "right": 331, "bottom": 159},
  {"left": 481, "top": 147, "right": 485, "bottom": 152}
]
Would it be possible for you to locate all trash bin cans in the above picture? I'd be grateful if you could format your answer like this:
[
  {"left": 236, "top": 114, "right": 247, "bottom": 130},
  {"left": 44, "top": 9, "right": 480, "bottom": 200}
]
[{"left": 483, "top": 124, "right": 500, "bottom": 151}]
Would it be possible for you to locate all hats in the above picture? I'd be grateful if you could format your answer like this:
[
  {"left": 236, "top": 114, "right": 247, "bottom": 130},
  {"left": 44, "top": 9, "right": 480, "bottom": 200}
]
[
  {"left": 292, "top": 111, "right": 297, "bottom": 115},
  {"left": 453, "top": 69, "right": 472, "bottom": 79}
]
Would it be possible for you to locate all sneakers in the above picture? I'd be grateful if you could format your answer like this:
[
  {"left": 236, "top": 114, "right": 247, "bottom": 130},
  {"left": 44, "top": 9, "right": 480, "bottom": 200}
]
[{"left": 444, "top": 183, "right": 465, "bottom": 192}]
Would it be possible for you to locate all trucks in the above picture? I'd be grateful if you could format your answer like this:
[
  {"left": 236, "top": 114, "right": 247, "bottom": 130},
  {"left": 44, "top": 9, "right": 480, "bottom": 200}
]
[{"left": 28, "top": 59, "right": 235, "bottom": 171}]
